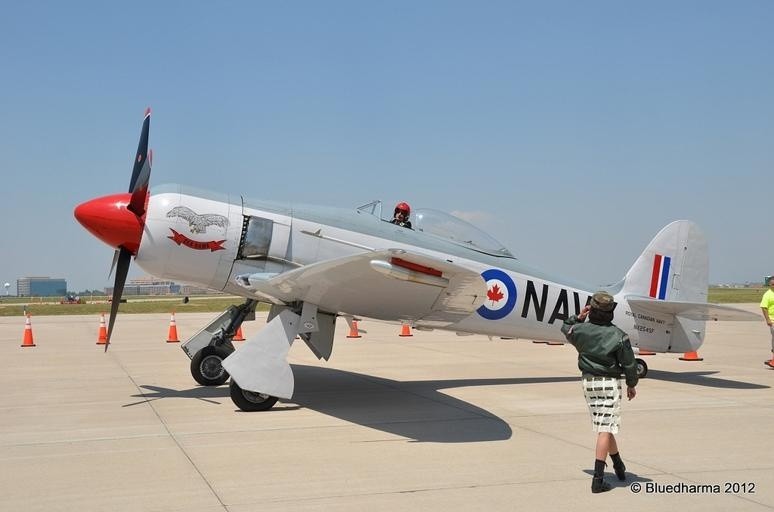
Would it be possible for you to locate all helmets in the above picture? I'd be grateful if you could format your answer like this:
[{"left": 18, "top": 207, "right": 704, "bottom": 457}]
[{"left": 393, "top": 202, "right": 411, "bottom": 221}]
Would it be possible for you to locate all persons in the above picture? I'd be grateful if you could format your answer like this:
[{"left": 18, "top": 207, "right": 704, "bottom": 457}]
[
  {"left": 391, "top": 202, "right": 412, "bottom": 229},
  {"left": 560, "top": 292, "right": 639, "bottom": 493},
  {"left": 759, "top": 276, "right": 774, "bottom": 367}
]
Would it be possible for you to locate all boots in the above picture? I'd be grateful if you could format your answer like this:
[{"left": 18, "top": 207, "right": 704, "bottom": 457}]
[
  {"left": 591, "top": 459, "right": 612, "bottom": 494},
  {"left": 609, "top": 452, "right": 627, "bottom": 482}
]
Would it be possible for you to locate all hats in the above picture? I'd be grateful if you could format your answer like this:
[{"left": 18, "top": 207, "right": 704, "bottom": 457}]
[{"left": 589, "top": 289, "right": 618, "bottom": 312}]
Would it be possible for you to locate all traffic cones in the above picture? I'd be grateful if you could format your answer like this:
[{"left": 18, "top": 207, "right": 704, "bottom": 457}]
[
  {"left": 95, "top": 313, "right": 111, "bottom": 345},
  {"left": 21, "top": 313, "right": 35, "bottom": 347},
  {"left": 233, "top": 325, "right": 246, "bottom": 341},
  {"left": 678, "top": 350, "right": 703, "bottom": 361},
  {"left": 501, "top": 337, "right": 565, "bottom": 345},
  {"left": 347, "top": 319, "right": 362, "bottom": 338},
  {"left": 638, "top": 349, "right": 656, "bottom": 355},
  {"left": 167, "top": 312, "right": 180, "bottom": 343},
  {"left": 769, "top": 354, "right": 774, "bottom": 367},
  {"left": 398, "top": 324, "right": 413, "bottom": 337}
]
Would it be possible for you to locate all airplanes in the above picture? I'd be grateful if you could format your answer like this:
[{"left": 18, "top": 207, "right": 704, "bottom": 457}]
[{"left": 74, "top": 106, "right": 765, "bottom": 412}]
